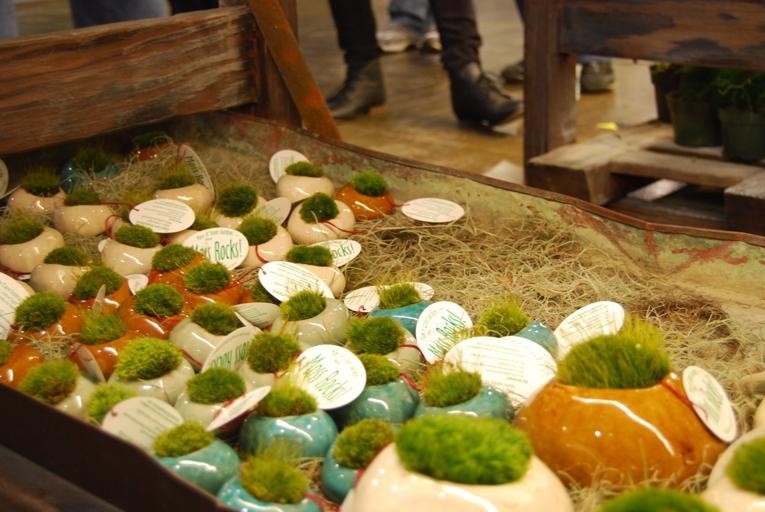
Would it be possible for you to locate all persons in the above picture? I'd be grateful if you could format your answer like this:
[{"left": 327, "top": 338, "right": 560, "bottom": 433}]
[
  {"left": 502, "top": 1, "right": 616, "bottom": 92},
  {"left": 375, "top": 1, "right": 442, "bottom": 53},
  {"left": 323, "top": 0, "right": 525, "bottom": 128}
]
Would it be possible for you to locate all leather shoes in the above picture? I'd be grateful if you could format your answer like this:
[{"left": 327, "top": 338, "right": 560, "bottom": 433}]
[
  {"left": 450, "top": 74, "right": 525, "bottom": 127},
  {"left": 322, "top": 75, "right": 385, "bottom": 118}
]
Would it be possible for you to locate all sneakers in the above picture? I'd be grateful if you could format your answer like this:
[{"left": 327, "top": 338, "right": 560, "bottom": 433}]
[
  {"left": 581, "top": 60, "right": 618, "bottom": 94},
  {"left": 502, "top": 62, "right": 527, "bottom": 83},
  {"left": 373, "top": 18, "right": 441, "bottom": 52}
]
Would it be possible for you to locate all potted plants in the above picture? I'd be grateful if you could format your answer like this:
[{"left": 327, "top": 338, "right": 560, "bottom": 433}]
[
  {"left": 716, "top": 67, "right": 765, "bottom": 164},
  {"left": 647, "top": 60, "right": 677, "bottom": 124},
  {"left": 664, "top": 65, "right": 720, "bottom": 149}
]
